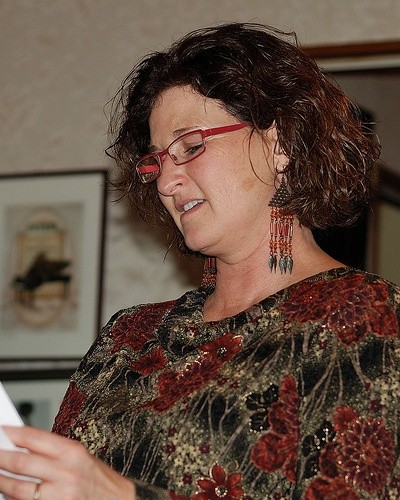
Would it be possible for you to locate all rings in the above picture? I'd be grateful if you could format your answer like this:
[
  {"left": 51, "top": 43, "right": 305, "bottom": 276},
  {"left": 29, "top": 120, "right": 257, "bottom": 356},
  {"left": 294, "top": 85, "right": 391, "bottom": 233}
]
[{"left": 31, "top": 483, "right": 42, "bottom": 499}]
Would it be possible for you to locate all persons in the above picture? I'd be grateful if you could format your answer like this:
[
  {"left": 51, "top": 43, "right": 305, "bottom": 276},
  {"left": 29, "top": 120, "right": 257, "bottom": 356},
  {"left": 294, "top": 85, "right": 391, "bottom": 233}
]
[{"left": 0, "top": 18, "right": 400, "bottom": 499}]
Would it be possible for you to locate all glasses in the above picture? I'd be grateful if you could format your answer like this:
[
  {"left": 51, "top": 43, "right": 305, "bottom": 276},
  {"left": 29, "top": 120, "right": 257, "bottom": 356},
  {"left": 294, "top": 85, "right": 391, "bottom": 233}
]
[{"left": 135, "top": 121, "right": 254, "bottom": 185}]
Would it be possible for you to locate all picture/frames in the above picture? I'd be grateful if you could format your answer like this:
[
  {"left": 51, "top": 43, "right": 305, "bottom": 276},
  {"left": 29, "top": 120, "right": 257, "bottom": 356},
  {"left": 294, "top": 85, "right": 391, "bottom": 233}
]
[{"left": 1, "top": 167, "right": 111, "bottom": 381}]
